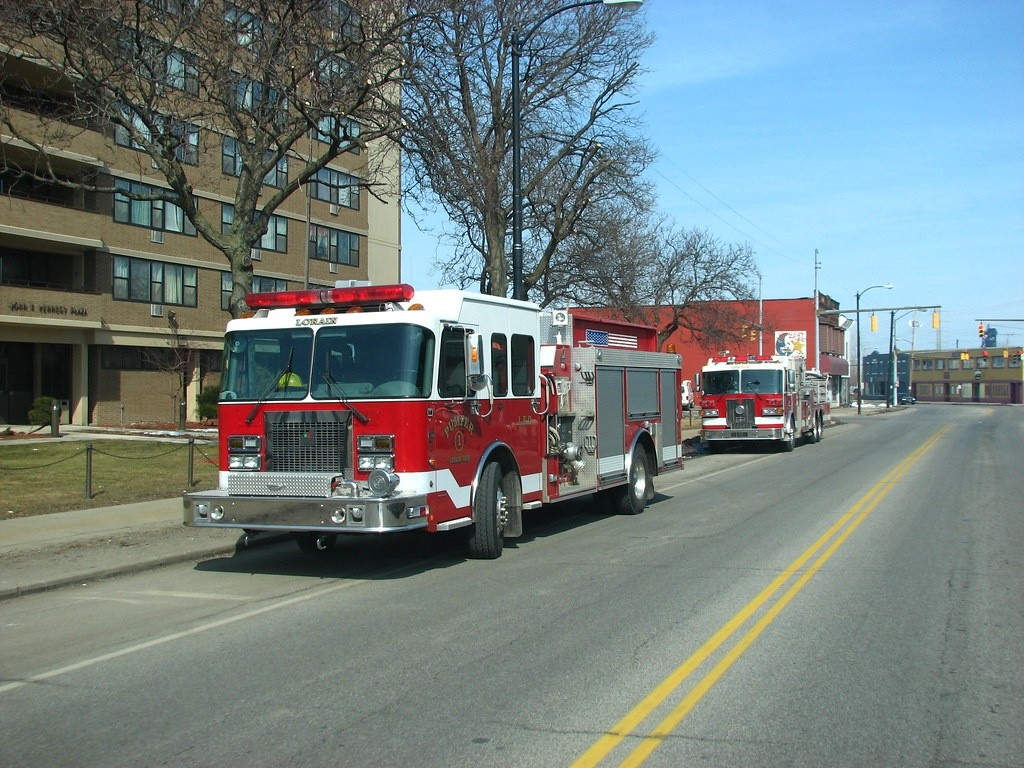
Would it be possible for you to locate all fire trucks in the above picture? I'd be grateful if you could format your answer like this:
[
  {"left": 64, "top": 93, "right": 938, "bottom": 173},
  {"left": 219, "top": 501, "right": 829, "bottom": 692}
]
[
  {"left": 694, "top": 355, "right": 831, "bottom": 452},
  {"left": 184, "top": 285, "right": 686, "bottom": 561}
]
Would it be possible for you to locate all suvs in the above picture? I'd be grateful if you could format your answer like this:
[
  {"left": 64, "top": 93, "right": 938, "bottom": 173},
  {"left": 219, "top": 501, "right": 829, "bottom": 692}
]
[{"left": 679, "top": 380, "right": 696, "bottom": 411}]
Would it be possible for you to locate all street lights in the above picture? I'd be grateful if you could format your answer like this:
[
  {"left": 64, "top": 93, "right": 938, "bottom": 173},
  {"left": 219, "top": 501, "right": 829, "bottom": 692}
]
[
  {"left": 856, "top": 284, "right": 893, "bottom": 415},
  {"left": 886, "top": 308, "right": 927, "bottom": 409}
]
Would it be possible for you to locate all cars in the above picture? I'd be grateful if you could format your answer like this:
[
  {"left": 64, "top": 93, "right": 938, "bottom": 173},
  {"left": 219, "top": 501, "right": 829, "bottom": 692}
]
[{"left": 889, "top": 392, "right": 916, "bottom": 405}]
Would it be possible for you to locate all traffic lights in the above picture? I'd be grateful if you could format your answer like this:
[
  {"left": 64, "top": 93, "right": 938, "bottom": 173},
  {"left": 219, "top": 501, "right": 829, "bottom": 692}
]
[
  {"left": 1016, "top": 347, "right": 1022, "bottom": 360},
  {"left": 983, "top": 351, "right": 988, "bottom": 361},
  {"left": 978, "top": 324, "right": 984, "bottom": 337},
  {"left": 1012, "top": 354, "right": 1016, "bottom": 364}
]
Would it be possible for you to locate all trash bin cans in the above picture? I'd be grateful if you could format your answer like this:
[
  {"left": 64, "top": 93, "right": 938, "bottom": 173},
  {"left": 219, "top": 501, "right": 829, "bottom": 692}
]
[{"left": 59, "top": 400, "right": 69, "bottom": 425}]
